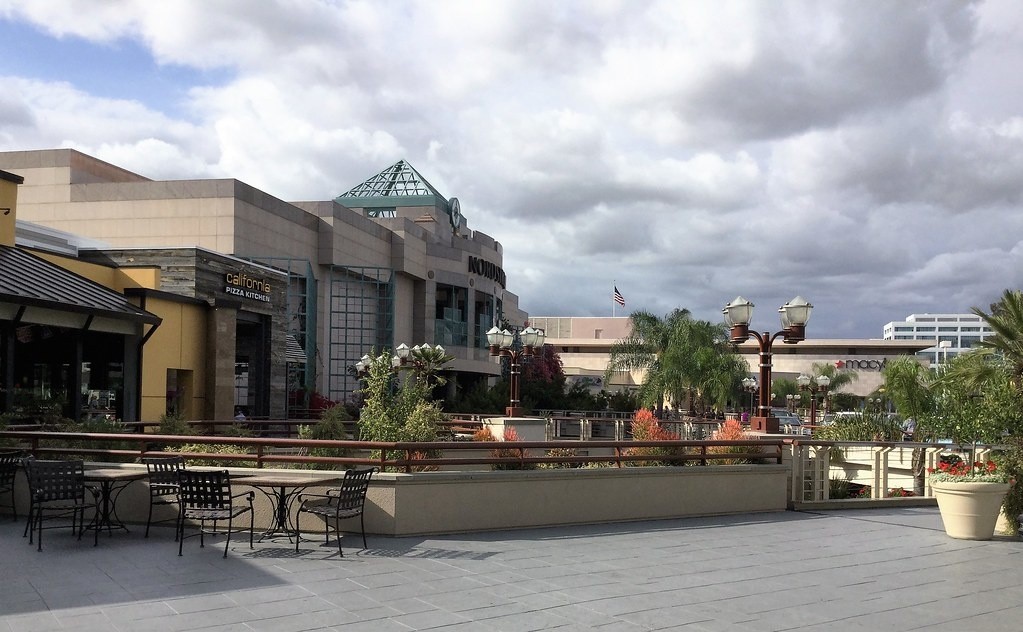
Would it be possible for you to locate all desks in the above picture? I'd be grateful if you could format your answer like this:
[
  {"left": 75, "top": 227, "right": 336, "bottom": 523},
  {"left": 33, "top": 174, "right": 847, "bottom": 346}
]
[
  {"left": 224, "top": 473, "right": 342, "bottom": 544},
  {"left": 51, "top": 469, "right": 149, "bottom": 537}
]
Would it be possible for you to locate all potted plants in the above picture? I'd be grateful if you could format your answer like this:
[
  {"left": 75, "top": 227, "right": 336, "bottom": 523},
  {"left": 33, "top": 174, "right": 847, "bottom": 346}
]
[{"left": 929, "top": 348, "right": 1023, "bottom": 540}]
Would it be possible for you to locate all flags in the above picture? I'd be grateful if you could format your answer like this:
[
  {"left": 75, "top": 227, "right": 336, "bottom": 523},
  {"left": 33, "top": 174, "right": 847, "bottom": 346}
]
[{"left": 615, "top": 286, "right": 625, "bottom": 307}]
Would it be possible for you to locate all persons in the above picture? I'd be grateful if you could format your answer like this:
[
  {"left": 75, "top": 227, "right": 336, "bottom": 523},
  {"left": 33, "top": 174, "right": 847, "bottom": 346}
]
[
  {"left": 665, "top": 406, "right": 670, "bottom": 419},
  {"left": 705, "top": 409, "right": 725, "bottom": 420},
  {"left": 902, "top": 418, "right": 915, "bottom": 441},
  {"left": 742, "top": 410, "right": 749, "bottom": 422},
  {"left": 234, "top": 407, "right": 246, "bottom": 420}
]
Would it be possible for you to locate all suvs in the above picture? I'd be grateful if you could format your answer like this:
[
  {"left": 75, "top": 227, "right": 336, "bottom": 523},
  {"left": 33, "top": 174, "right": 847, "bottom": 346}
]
[{"left": 771, "top": 409, "right": 792, "bottom": 417}]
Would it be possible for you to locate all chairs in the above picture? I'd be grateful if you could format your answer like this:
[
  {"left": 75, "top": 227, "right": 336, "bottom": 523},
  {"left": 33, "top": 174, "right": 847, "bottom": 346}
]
[
  {"left": 141, "top": 457, "right": 186, "bottom": 542},
  {"left": 295, "top": 467, "right": 375, "bottom": 557},
  {"left": 176, "top": 468, "right": 255, "bottom": 559},
  {"left": 21, "top": 453, "right": 84, "bottom": 546},
  {"left": 0, "top": 450, "right": 23, "bottom": 523},
  {"left": 35, "top": 458, "right": 85, "bottom": 552}
]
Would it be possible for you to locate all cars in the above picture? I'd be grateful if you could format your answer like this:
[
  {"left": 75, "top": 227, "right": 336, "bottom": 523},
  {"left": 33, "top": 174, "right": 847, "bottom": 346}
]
[
  {"left": 792, "top": 413, "right": 804, "bottom": 424},
  {"left": 773, "top": 417, "right": 806, "bottom": 434},
  {"left": 904, "top": 428, "right": 916, "bottom": 440}
]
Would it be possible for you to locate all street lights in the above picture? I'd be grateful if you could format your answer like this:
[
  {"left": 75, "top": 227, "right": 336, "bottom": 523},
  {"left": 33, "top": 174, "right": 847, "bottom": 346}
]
[
  {"left": 356, "top": 342, "right": 446, "bottom": 407},
  {"left": 741, "top": 377, "right": 800, "bottom": 417},
  {"left": 722, "top": 293, "right": 815, "bottom": 432},
  {"left": 796, "top": 374, "right": 830, "bottom": 433},
  {"left": 940, "top": 341, "right": 952, "bottom": 397},
  {"left": 868, "top": 398, "right": 881, "bottom": 415},
  {"left": 486, "top": 325, "right": 547, "bottom": 418}
]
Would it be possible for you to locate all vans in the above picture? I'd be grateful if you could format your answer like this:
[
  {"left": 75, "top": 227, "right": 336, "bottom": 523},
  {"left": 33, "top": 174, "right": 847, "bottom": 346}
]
[{"left": 831, "top": 411, "right": 863, "bottom": 427}]
